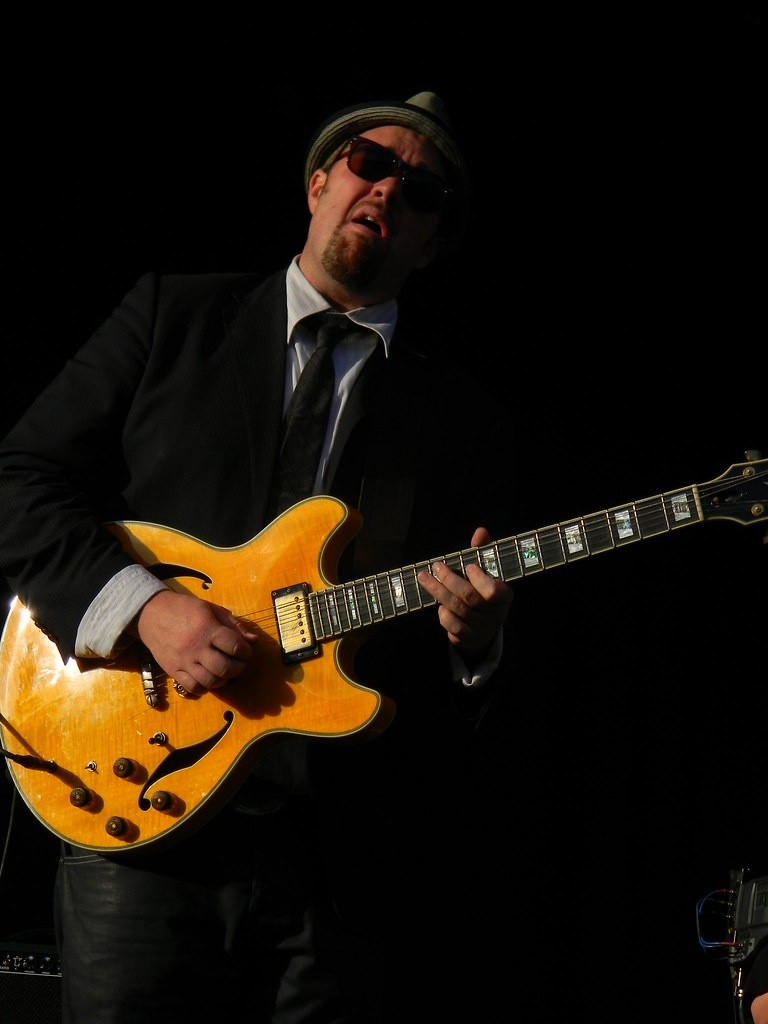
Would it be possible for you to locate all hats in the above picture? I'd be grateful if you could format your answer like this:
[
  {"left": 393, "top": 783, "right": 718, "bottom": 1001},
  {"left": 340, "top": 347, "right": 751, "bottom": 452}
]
[{"left": 304, "top": 91, "right": 468, "bottom": 228}]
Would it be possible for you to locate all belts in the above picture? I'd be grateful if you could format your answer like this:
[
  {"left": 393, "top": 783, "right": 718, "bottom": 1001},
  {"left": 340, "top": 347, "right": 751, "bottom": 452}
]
[{"left": 229, "top": 768, "right": 330, "bottom": 815}]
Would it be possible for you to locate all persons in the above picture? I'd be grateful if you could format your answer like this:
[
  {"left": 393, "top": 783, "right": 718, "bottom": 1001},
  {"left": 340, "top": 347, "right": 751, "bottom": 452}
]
[{"left": 0, "top": 92, "right": 511, "bottom": 1024}]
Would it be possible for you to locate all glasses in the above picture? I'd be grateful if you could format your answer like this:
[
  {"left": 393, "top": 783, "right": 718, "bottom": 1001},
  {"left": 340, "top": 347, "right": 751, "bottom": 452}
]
[{"left": 339, "top": 130, "right": 456, "bottom": 218}]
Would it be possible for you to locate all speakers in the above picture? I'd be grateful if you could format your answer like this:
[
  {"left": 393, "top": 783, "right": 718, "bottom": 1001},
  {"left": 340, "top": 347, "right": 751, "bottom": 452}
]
[{"left": 0, "top": 940, "right": 64, "bottom": 1024}]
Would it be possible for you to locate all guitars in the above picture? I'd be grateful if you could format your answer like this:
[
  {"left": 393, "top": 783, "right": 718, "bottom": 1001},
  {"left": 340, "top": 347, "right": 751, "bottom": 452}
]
[{"left": 0, "top": 449, "right": 767, "bottom": 860}]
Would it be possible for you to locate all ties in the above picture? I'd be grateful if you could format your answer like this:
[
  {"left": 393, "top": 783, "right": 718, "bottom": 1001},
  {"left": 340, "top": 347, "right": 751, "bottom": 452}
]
[{"left": 261, "top": 313, "right": 363, "bottom": 529}]
